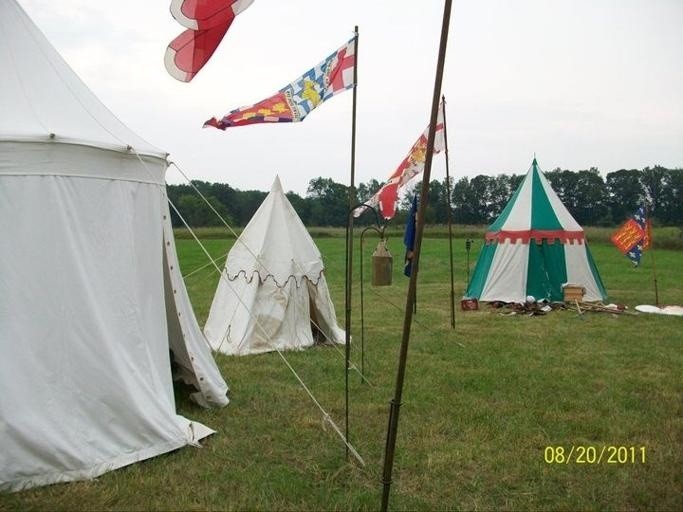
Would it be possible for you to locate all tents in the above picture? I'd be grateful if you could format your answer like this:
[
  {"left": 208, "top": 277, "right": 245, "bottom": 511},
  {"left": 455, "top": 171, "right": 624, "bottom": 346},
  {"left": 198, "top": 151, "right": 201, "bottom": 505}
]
[
  {"left": 0, "top": 0, "right": 230, "bottom": 496},
  {"left": 462, "top": 152, "right": 607, "bottom": 304}
]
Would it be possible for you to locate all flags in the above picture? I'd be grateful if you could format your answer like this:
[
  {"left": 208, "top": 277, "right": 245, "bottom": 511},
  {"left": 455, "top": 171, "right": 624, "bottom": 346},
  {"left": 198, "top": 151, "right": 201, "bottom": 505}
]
[
  {"left": 203, "top": 172, "right": 353, "bottom": 358},
  {"left": 611, "top": 198, "right": 652, "bottom": 269},
  {"left": 403, "top": 195, "right": 418, "bottom": 277}
]
